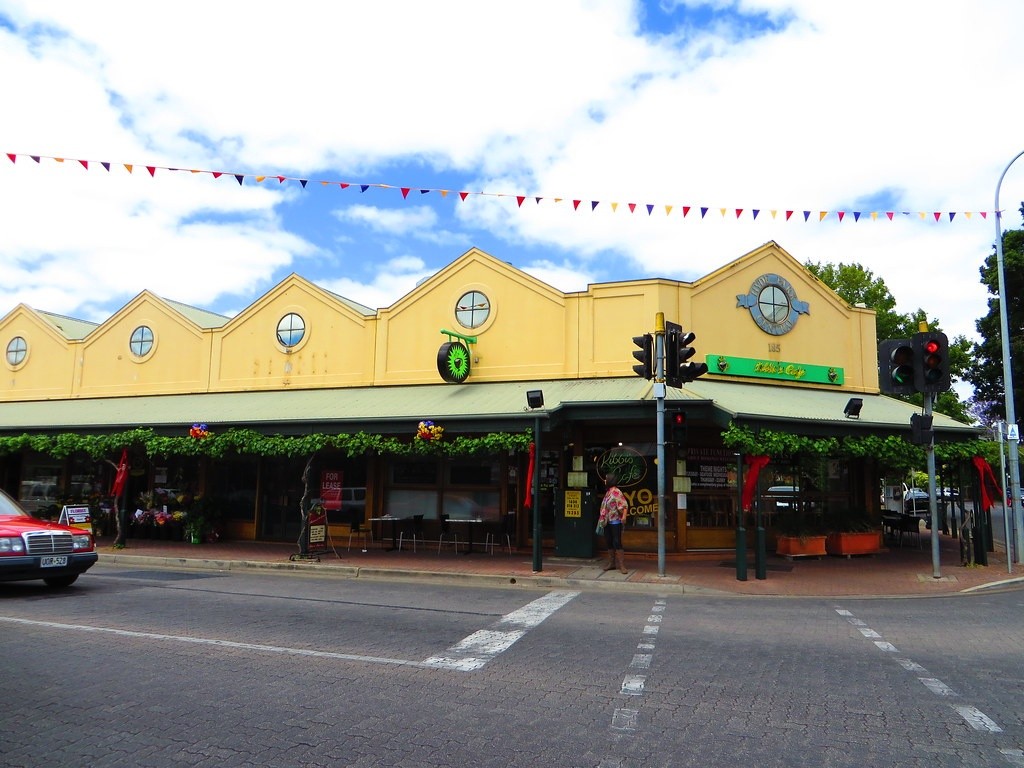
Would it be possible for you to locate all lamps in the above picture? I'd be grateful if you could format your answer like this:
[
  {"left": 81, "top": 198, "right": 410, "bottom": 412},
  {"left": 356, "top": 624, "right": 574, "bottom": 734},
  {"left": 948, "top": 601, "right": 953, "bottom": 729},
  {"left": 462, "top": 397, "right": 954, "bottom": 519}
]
[
  {"left": 526, "top": 388, "right": 544, "bottom": 411},
  {"left": 843, "top": 397, "right": 863, "bottom": 420}
]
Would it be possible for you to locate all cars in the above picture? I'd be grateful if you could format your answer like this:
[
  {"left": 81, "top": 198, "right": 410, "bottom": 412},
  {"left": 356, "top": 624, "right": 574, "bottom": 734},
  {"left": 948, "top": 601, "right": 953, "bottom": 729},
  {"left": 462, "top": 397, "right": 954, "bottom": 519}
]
[
  {"left": 904, "top": 486, "right": 960, "bottom": 509},
  {"left": 757, "top": 485, "right": 816, "bottom": 513},
  {"left": 0, "top": 489, "right": 98, "bottom": 589},
  {"left": 1007, "top": 486, "right": 1024, "bottom": 506}
]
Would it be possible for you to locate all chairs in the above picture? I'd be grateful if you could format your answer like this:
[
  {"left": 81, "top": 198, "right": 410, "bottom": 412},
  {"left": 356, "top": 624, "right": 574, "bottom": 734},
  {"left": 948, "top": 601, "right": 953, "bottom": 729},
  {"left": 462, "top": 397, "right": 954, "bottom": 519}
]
[
  {"left": 882, "top": 509, "right": 923, "bottom": 552},
  {"left": 438, "top": 514, "right": 465, "bottom": 555},
  {"left": 484, "top": 514, "right": 513, "bottom": 556},
  {"left": 347, "top": 509, "right": 374, "bottom": 552},
  {"left": 398, "top": 514, "right": 426, "bottom": 554}
]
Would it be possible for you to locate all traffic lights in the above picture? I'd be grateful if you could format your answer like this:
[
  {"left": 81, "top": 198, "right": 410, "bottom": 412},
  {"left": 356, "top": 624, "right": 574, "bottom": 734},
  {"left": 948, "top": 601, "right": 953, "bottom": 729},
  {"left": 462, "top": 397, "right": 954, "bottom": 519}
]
[
  {"left": 878, "top": 338, "right": 917, "bottom": 396},
  {"left": 632, "top": 332, "right": 652, "bottom": 380},
  {"left": 665, "top": 320, "right": 708, "bottom": 388},
  {"left": 913, "top": 330, "right": 951, "bottom": 393}
]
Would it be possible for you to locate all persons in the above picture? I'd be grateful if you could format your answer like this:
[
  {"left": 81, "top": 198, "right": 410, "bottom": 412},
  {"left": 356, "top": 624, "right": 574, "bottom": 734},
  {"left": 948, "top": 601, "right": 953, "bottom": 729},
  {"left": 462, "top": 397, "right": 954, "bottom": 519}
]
[{"left": 598, "top": 473, "right": 628, "bottom": 573}]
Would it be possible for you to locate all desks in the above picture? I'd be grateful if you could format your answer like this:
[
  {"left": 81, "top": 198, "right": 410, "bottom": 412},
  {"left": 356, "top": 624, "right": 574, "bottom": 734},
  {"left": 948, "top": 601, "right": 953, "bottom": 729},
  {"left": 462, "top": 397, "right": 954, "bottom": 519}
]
[
  {"left": 884, "top": 516, "right": 917, "bottom": 547},
  {"left": 445, "top": 517, "right": 489, "bottom": 556},
  {"left": 368, "top": 515, "right": 410, "bottom": 552}
]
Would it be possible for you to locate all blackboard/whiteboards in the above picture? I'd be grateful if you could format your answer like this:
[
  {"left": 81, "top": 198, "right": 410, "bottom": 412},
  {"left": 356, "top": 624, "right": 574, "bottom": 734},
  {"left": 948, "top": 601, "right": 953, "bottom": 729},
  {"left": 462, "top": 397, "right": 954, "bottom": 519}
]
[{"left": 597, "top": 446, "right": 648, "bottom": 487}]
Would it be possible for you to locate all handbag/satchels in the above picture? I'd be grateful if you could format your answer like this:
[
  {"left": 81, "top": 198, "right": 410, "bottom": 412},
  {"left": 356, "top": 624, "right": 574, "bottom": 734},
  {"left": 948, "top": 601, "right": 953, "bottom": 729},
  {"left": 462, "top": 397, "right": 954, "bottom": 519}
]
[{"left": 596, "top": 524, "right": 605, "bottom": 536}]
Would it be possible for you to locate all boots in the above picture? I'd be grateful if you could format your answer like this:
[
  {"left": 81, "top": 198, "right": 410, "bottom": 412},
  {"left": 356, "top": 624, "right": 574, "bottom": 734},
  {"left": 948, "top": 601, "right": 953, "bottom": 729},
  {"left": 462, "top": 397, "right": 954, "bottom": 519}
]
[
  {"left": 603, "top": 549, "right": 616, "bottom": 571},
  {"left": 616, "top": 549, "right": 628, "bottom": 574}
]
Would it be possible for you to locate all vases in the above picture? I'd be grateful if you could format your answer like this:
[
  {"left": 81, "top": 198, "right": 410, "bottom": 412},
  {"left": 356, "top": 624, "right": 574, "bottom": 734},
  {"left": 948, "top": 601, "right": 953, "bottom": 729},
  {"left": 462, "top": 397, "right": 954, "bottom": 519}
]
[
  {"left": 159, "top": 526, "right": 171, "bottom": 540},
  {"left": 190, "top": 522, "right": 203, "bottom": 545},
  {"left": 127, "top": 528, "right": 136, "bottom": 538},
  {"left": 171, "top": 527, "right": 183, "bottom": 541},
  {"left": 136, "top": 528, "right": 150, "bottom": 539},
  {"left": 150, "top": 527, "right": 159, "bottom": 539}
]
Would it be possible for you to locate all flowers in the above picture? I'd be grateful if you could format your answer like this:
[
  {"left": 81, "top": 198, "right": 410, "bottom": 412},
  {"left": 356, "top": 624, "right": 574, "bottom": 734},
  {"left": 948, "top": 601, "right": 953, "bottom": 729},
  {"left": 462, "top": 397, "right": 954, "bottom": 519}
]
[
  {"left": 170, "top": 511, "right": 188, "bottom": 527},
  {"left": 184, "top": 496, "right": 205, "bottom": 527},
  {"left": 153, "top": 487, "right": 176, "bottom": 510},
  {"left": 155, "top": 512, "right": 172, "bottom": 526},
  {"left": 140, "top": 489, "right": 154, "bottom": 510},
  {"left": 189, "top": 423, "right": 209, "bottom": 441},
  {"left": 413, "top": 420, "right": 444, "bottom": 445},
  {"left": 170, "top": 494, "right": 190, "bottom": 510},
  {"left": 137, "top": 512, "right": 151, "bottom": 527}
]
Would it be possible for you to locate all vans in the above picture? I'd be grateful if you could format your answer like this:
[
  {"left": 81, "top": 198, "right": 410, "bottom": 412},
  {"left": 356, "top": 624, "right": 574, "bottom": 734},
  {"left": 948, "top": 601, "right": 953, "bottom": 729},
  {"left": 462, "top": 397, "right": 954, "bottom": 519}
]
[{"left": 311, "top": 486, "right": 368, "bottom": 524}]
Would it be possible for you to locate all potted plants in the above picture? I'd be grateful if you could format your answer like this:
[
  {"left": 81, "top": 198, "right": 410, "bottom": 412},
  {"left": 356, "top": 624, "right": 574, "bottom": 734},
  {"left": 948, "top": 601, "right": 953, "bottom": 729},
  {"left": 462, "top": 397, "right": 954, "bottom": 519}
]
[
  {"left": 822, "top": 506, "right": 881, "bottom": 559},
  {"left": 200, "top": 495, "right": 226, "bottom": 544},
  {"left": 772, "top": 508, "right": 828, "bottom": 562}
]
